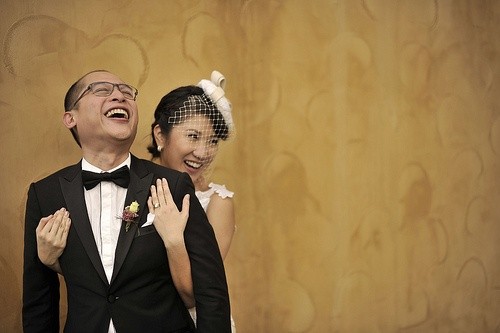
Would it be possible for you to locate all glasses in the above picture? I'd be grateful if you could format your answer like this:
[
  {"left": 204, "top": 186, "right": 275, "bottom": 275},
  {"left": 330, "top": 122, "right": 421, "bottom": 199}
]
[{"left": 70, "top": 82, "right": 138, "bottom": 110}]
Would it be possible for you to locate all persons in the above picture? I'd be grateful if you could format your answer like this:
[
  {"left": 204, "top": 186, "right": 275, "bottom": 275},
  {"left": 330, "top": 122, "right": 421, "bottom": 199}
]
[
  {"left": 20, "top": 69, "right": 233, "bottom": 333},
  {"left": 35, "top": 70, "right": 236, "bottom": 333}
]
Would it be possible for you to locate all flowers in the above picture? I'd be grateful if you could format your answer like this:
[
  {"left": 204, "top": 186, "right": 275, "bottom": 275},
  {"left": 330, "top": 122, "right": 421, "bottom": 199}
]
[{"left": 114, "top": 199, "right": 139, "bottom": 233}]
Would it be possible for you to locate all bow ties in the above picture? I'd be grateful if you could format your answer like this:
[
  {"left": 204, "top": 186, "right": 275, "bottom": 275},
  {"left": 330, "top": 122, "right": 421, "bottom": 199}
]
[{"left": 81, "top": 165, "right": 130, "bottom": 191}]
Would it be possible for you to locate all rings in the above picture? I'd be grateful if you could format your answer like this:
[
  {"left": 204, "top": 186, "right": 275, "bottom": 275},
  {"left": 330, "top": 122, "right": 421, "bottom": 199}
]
[{"left": 153, "top": 203, "right": 160, "bottom": 209}]
